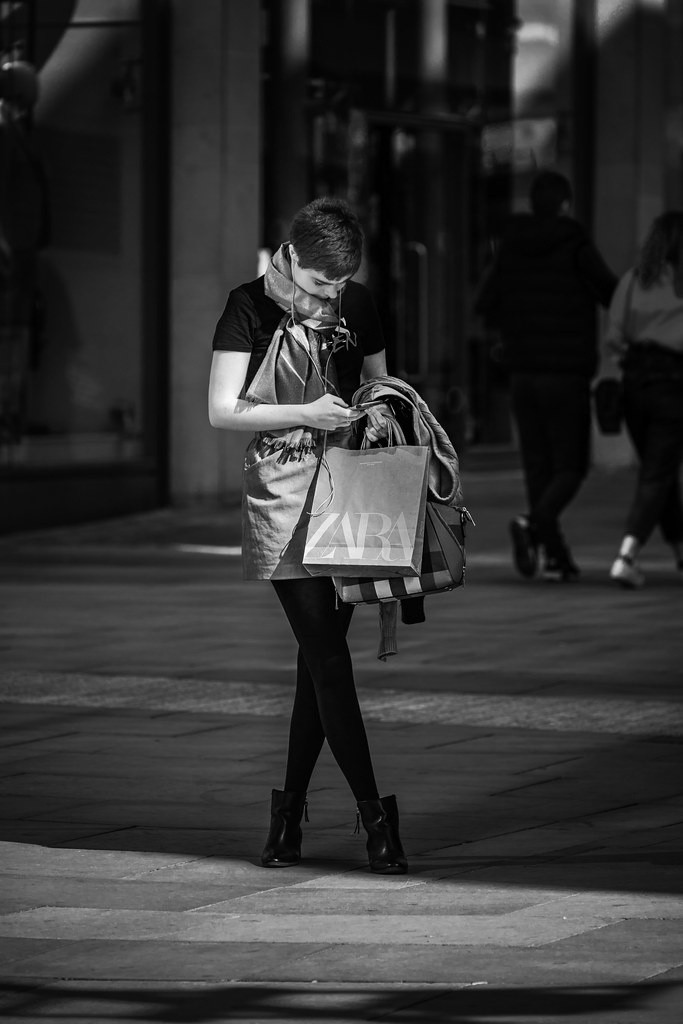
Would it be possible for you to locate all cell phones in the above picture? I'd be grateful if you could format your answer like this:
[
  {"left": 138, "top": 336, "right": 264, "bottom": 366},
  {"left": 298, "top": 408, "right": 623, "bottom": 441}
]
[{"left": 349, "top": 400, "right": 383, "bottom": 411}]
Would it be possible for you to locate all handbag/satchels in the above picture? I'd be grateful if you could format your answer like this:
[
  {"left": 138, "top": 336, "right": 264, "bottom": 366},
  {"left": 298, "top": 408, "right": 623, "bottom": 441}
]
[
  {"left": 301, "top": 415, "right": 431, "bottom": 577},
  {"left": 595, "top": 378, "right": 622, "bottom": 435},
  {"left": 332, "top": 414, "right": 466, "bottom": 605}
]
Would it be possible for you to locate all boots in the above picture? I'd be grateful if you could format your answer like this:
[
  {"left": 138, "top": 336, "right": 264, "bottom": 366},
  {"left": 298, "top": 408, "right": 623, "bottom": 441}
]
[
  {"left": 354, "top": 795, "right": 409, "bottom": 872},
  {"left": 261, "top": 789, "right": 309, "bottom": 865}
]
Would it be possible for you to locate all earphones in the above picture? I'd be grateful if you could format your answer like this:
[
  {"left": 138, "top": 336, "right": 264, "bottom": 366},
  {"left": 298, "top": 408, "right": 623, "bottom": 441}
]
[{"left": 291, "top": 254, "right": 297, "bottom": 264}]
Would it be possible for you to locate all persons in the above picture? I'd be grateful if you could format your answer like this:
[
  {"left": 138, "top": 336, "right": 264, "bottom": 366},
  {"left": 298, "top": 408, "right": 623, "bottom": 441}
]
[
  {"left": 207, "top": 198, "right": 407, "bottom": 874},
  {"left": 471, "top": 172, "right": 615, "bottom": 584},
  {"left": 601, "top": 209, "right": 683, "bottom": 588}
]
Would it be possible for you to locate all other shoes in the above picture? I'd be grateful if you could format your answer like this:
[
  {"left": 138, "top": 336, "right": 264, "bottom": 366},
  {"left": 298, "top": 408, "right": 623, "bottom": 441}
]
[
  {"left": 610, "top": 559, "right": 645, "bottom": 584},
  {"left": 545, "top": 547, "right": 582, "bottom": 573},
  {"left": 510, "top": 514, "right": 539, "bottom": 575}
]
